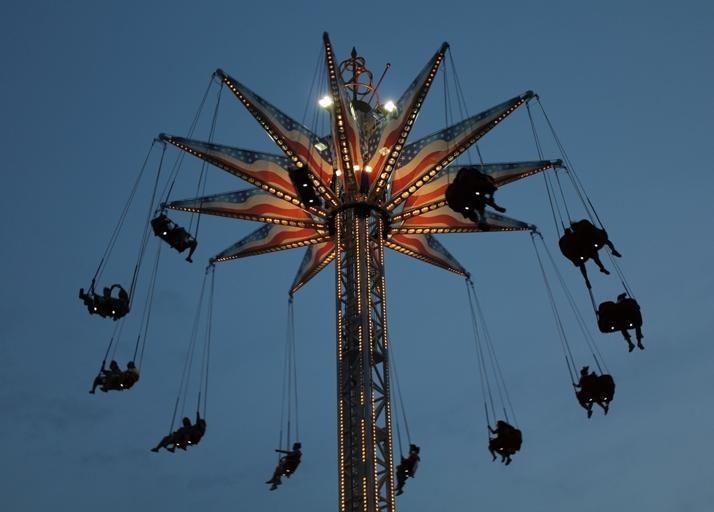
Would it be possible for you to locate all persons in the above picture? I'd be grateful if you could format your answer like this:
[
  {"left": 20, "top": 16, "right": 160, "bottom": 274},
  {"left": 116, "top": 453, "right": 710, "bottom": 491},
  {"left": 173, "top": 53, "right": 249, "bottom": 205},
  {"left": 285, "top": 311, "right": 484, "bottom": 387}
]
[
  {"left": 615, "top": 291, "right": 646, "bottom": 352},
  {"left": 454, "top": 165, "right": 506, "bottom": 212},
  {"left": 563, "top": 227, "right": 596, "bottom": 291},
  {"left": 571, "top": 219, "right": 622, "bottom": 276},
  {"left": 488, "top": 419, "right": 503, "bottom": 463},
  {"left": 440, "top": 184, "right": 478, "bottom": 224},
  {"left": 170, "top": 219, "right": 197, "bottom": 263},
  {"left": 78, "top": 282, "right": 129, "bottom": 314},
  {"left": 500, "top": 453, "right": 511, "bottom": 468},
  {"left": 87, "top": 360, "right": 123, "bottom": 395},
  {"left": 266, "top": 441, "right": 302, "bottom": 491},
  {"left": 570, "top": 365, "right": 610, "bottom": 419},
  {"left": 126, "top": 357, "right": 141, "bottom": 374},
  {"left": 151, "top": 417, "right": 193, "bottom": 452},
  {"left": 395, "top": 442, "right": 417, "bottom": 497}
]
